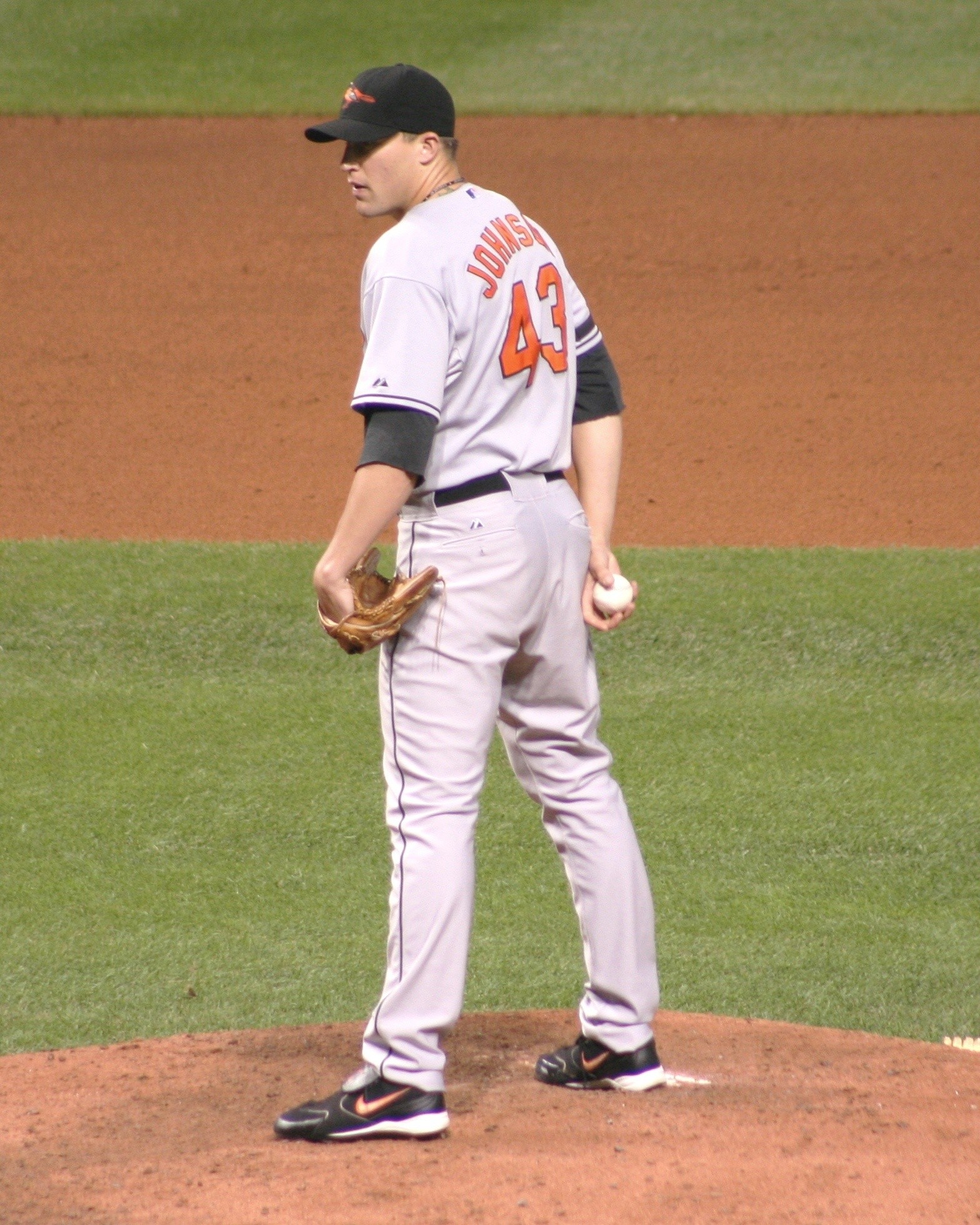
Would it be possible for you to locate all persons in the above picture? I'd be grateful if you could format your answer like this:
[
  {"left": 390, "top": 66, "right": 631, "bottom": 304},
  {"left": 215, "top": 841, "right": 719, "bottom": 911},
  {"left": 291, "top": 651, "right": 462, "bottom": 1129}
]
[{"left": 272, "top": 66, "right": 664, "bottom": 1140}]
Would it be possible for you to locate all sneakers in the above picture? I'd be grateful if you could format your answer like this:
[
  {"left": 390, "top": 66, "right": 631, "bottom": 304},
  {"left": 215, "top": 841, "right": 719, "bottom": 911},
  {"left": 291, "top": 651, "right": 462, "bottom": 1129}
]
[
  {"left": 537, "top": 1034, "right": 665, "bottom": 1094},
  {"left": 276, "top": 1062, "right": 451, "bottom": 1144}
]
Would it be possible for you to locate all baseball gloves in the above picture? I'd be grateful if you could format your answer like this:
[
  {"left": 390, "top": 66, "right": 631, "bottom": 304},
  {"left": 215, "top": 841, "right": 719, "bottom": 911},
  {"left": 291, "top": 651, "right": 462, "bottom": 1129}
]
[{"left": 315, "top": 547, "right": 439, "bottom": 655}]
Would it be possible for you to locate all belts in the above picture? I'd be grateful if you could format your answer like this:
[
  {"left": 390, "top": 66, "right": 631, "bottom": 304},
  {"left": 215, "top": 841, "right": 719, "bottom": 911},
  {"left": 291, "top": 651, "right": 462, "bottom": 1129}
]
[{"left": 435, "top": 464, "right": 566, "bottom": 509}]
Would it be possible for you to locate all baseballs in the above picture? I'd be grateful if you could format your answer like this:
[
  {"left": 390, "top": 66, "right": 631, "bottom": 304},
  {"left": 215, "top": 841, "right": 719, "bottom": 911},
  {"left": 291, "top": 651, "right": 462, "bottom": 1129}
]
[{"left": 593, "top": 573, "right": 633, "bottom": 615}]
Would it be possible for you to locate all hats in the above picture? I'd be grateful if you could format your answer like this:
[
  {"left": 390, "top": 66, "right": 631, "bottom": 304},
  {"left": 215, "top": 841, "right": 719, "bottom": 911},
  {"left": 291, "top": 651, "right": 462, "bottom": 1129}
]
[{"left": 301, "top": 62, "right": 454, "bottom": 143}]
